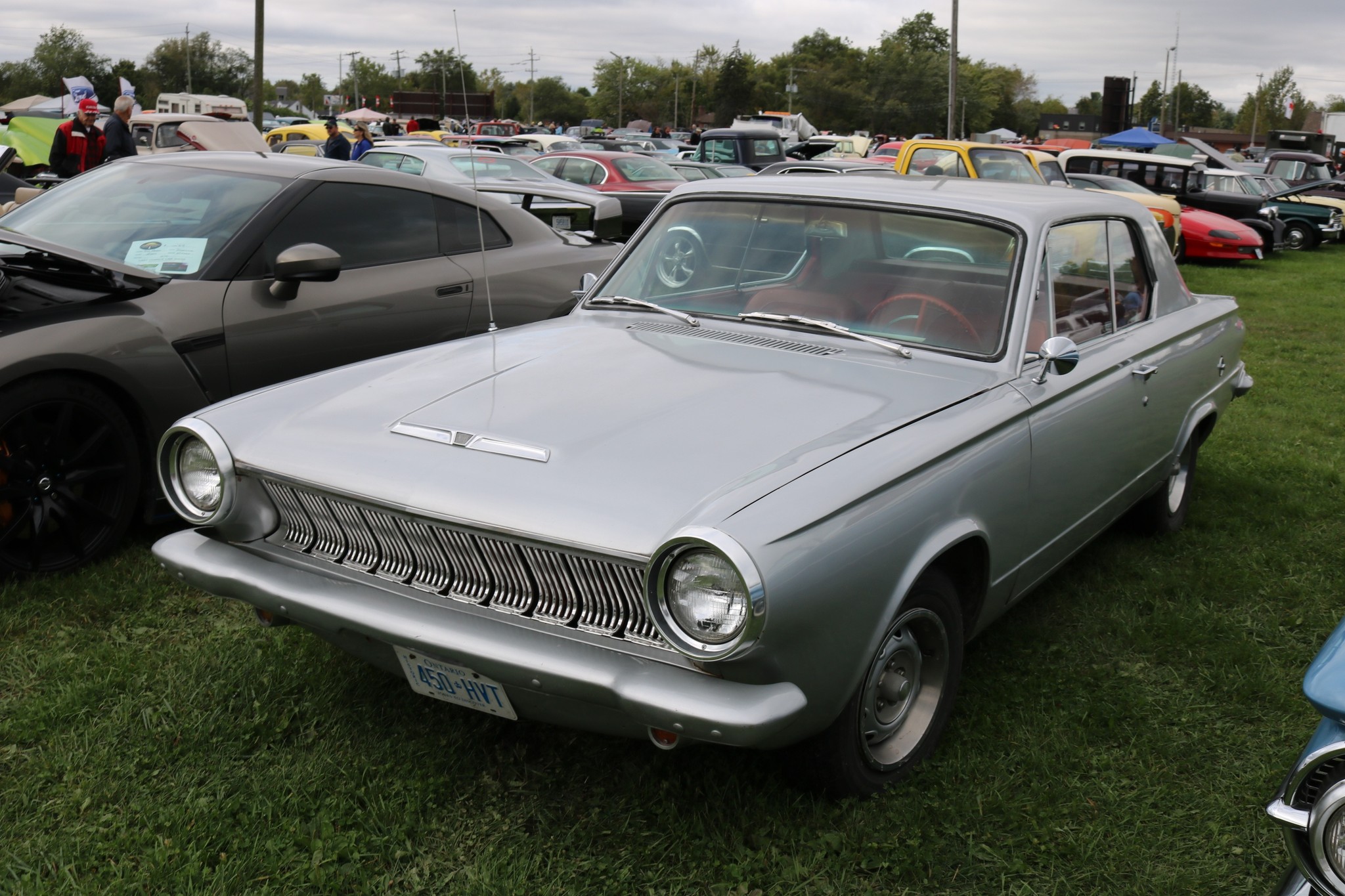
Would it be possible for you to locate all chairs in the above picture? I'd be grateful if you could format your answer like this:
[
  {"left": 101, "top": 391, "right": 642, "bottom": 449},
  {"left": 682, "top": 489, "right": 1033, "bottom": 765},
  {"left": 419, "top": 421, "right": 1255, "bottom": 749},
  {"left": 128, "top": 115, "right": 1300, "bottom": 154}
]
[
  {"left": 0, "top": 187, "right": 45, "bottom": 217},
  {"left": 565, "top": 165, "right": 587, "bottom": 185}
]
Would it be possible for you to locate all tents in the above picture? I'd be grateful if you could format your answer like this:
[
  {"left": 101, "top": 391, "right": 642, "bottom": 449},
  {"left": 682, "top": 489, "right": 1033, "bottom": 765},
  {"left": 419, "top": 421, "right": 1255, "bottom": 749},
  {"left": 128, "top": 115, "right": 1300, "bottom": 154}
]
[
  {"left": 1097, "top": 128, "right": 1177, "bottom": 153},
  {"left": 336, "top": 106, "right": 393, "bottom": 123}
]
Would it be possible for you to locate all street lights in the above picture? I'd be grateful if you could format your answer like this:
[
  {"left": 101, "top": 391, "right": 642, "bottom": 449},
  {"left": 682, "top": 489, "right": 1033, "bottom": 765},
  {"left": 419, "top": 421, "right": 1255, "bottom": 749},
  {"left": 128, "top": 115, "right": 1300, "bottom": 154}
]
[{"left": 1159, "top": 47, "right": 1176, "bottom": 136}]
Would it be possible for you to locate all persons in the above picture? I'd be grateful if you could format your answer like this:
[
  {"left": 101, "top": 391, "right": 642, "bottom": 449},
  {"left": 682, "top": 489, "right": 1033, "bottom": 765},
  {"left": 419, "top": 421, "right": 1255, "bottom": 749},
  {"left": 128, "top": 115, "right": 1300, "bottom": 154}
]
[
  {"left": 432, "top": 114, "right": 572, "bottom": 149},
  {"left": 49, "top": 99, "right": 106, "bottom": 185},
  {"left": 1085, "top": 131, "right": 1345, "bottom": 173},
  {"left": 99, "top": 95, "right": 138, "bottom": 166},
  {"left": 369, "top": 116, "right": 420, "bottom": 141},
  {"left": 590, "top": 117, "right": 702, "bottom": 145},
  {"left": 810, "top": 128, "right": 1046, "bottom": 181},
  {"left": 323, "top": 118, "right": 351, "bottom": 161},
  {"left": 350, "top": 121, "right": 374, "bottom": 161}
]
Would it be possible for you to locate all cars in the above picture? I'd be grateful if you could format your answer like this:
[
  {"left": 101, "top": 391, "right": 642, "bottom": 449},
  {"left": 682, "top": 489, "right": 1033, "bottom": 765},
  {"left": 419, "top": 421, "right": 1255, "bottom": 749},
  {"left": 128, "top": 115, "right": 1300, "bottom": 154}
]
[
  {"left": 353, "top": 145, "right": 622, "bottom": 247},
  {"left": 1263, "top": 619, "right": 1345, "bottom": 896},
  {"left": 661, "top": 158, "right": 757, "bottom": 184},
  {"left": 120, "top": 91, "right": 1345, "bottom": 291},
  {"left": 528, "top": 149, "right": 688, "bottom": 237},
  {"left": 1, "top": 140, "right": 628, "bottom": 582},
  {"left": 146, "top": 172, "right": 1255, "bottom": 801}
]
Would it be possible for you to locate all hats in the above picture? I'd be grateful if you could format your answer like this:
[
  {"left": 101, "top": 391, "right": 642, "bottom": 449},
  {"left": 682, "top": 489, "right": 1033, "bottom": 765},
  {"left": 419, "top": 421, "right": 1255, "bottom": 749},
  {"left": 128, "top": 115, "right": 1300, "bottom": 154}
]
[
  {"left": 538, "top": 121, "right": 543, "bottom": 125},
  {"left": 324, "top": 119, "right": 337, "bottom": 126},
  {"left": 79, "top": 99, "right": 100, "bottom": 114}
]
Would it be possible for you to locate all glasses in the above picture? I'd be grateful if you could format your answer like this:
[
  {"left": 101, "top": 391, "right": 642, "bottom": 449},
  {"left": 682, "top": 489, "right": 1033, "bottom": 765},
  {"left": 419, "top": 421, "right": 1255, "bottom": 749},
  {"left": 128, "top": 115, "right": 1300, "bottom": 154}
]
[
  {"left": 354, "top": 129, "right": 360, "bottom": 132},
  {"left": 326, "top": 126, "right": 333, "bottom": 129}
]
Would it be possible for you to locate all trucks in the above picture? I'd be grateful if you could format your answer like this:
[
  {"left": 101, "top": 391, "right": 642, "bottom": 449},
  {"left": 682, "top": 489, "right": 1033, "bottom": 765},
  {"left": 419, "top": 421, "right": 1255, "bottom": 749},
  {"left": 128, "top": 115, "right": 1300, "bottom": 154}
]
[{"left": 1301, "top": 109, "right": 1345, "bottom": 161}]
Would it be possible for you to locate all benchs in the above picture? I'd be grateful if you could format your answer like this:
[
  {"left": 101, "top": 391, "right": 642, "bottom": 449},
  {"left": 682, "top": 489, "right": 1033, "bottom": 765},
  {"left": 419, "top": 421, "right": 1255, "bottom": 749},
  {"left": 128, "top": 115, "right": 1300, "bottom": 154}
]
[{"left": 746, "top": 258, "right": 1119, "bottom": 349}]
